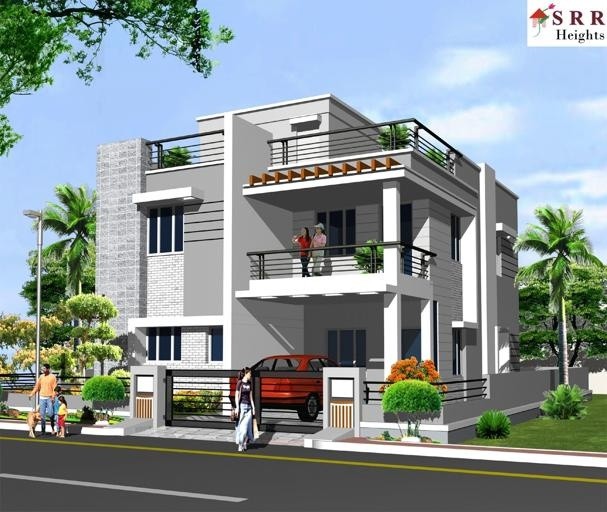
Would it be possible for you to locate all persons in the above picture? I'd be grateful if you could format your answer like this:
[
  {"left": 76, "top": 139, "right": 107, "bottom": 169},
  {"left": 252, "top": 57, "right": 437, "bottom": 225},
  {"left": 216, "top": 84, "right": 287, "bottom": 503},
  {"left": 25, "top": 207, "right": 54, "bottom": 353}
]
[
  {"left": 234, "top": 366, "right": 256, "bottom": 452},
  {"left": 291, "top": 227, "right": 312, "bottom": 277},
  {"left": 58, "top": 396, "right": 68, "bottom": 438},
  {"left": 29, "top": 364, "right": 57, "bottom": 435},
  {"left": 307, "top": 223, "right": 326, "bottom": 276},
  {"left": 54, "top": 386, "right": 62, "bottom": 437}
]
